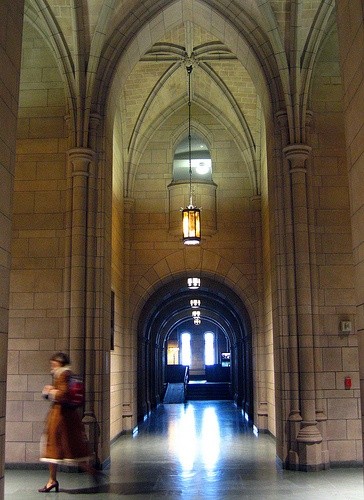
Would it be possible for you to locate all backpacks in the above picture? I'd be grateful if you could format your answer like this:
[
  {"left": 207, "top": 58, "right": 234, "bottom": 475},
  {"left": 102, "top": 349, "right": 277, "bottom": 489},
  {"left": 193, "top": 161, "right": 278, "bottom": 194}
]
[{"left": 66, "top": 373, "right": 85, "bottom": 407}]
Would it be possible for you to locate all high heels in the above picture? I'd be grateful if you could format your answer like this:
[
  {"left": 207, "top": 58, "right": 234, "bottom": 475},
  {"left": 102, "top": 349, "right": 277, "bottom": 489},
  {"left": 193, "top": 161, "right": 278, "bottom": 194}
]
[
  {"left": 92, "top": 471, "right": 106, "bottom": 490},
  {"left": 39, "top": 481, "right": 60, "bottom": 493}
]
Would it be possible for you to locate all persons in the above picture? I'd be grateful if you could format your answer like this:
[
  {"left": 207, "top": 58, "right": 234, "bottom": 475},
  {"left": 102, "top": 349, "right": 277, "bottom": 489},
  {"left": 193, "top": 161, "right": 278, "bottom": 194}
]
[{"left": 36, "top": 353, "right": 104, "bottom": 494}]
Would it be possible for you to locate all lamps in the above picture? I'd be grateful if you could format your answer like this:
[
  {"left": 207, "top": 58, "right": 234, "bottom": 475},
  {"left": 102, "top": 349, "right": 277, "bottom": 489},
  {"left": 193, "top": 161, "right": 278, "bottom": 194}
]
[
  {"left": 191, "top": 310, "right": 201, "bottom": 319},
  {"left": 193, "top": 319, "right": 202, "bottom": 325},
  {"left": 181, "top": 64, "right": 202, "bottom": 246},
  {"left": 187, "top": 277, "right": 201, "bottom": 290},
  {"left": 189, "top": 299, "right": 201, "bottom": 309}
]
